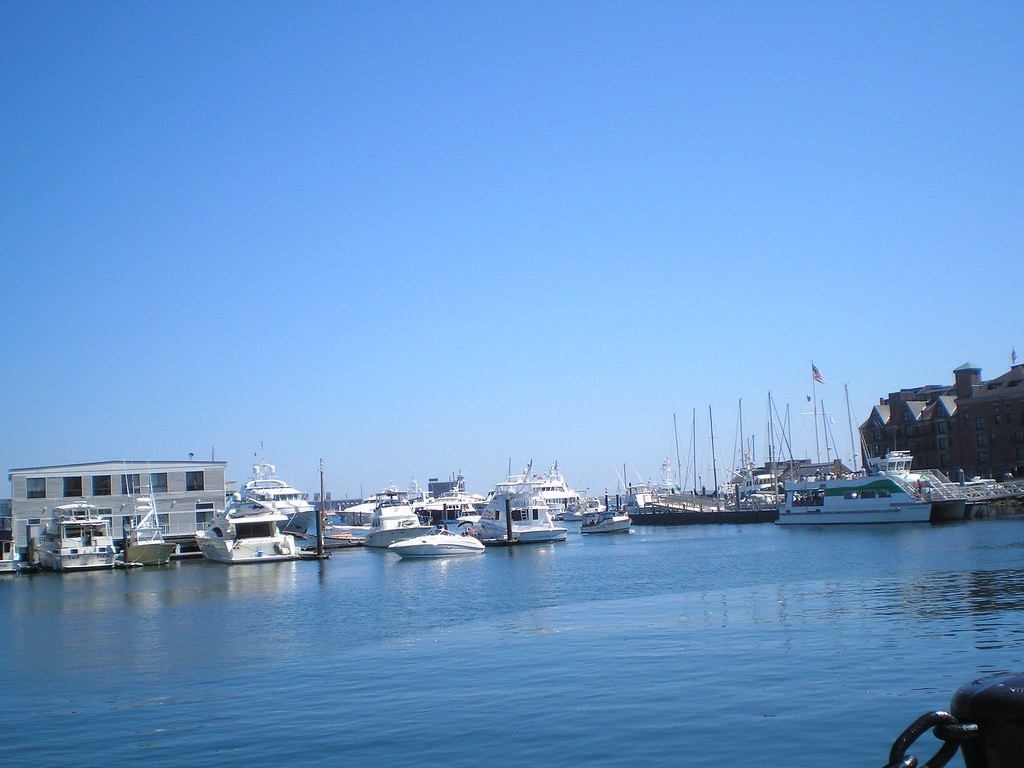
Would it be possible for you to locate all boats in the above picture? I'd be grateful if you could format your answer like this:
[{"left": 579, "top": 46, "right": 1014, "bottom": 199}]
[
  {"left": 119, "top": 454, "right": 177, "bottom": 566},
  {"left": 333, "top": 467, "right": 632, "bottom": 556},
  {"left": 243, "top": 439, "right": 314, "bottom": 531},
  {"left": 32, "top": 503, "right": 116, "bottom": 570},
  {"left": 194, "top": 491, "right": 302, "bottom": 563},
  {"left": 307, "top": 533, "right": 365, "bottom": 547}
]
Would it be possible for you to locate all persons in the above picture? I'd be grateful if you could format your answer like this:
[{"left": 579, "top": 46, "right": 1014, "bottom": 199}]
[
  {"left": 915, "top": 480, "right": 922, "bottom": 498},
  {"left": 793, "top": 493, "right": 823, "bottom": 507},
  {"left": 439, "top": 522, "right": 447, "bottom": 535},
  {"left": 590, "top": 520, "right": 595, "bottom": 525},
  {"left": 745, "top": 496, "right": 750, "bottom": 510},
  {"left": 1004, "top": 471, "right": 1013, "bottom": 482},
  {"left": 797, "top": 464, "right": 878, "bottom": 481}
]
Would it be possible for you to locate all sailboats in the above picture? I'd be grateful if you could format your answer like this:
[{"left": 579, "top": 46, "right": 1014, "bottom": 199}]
[{"left": 625, "top": 384, "right": 967, "bottom": 525}]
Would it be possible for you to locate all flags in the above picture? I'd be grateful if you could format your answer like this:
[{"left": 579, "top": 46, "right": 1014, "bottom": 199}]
[{"left": 812, "top": 364, "right": 825, "bottom": 384}]
